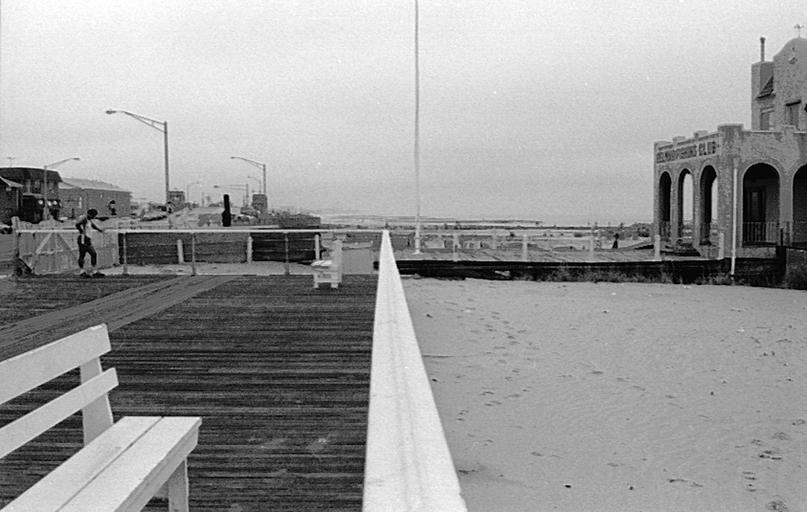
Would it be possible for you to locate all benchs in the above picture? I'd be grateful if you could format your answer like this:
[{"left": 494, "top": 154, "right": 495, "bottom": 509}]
[
  {"left": 310, "top": 239, "right": 343, "bottom": 290},
  {"left": 0, "top": 321, "right": 202, "bottom": 512}
]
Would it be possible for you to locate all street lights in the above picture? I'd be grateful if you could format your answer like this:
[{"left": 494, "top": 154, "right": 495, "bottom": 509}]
[
  {"left": 44, "top": 158, "right": 80, "bottom": 218},
  {"left": 187, "top": 181, "right": 200, "bottom": 202},
  {"left": 214, "top": 156, "right": 267, "bottom": 205},
  {"left": 106, "top": 109, "right": 169, "bottom": 201}
]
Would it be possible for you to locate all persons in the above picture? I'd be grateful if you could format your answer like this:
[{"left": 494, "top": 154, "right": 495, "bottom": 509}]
[{"left": 75, "top": 209, "right": 103, "bottom": 276}]
